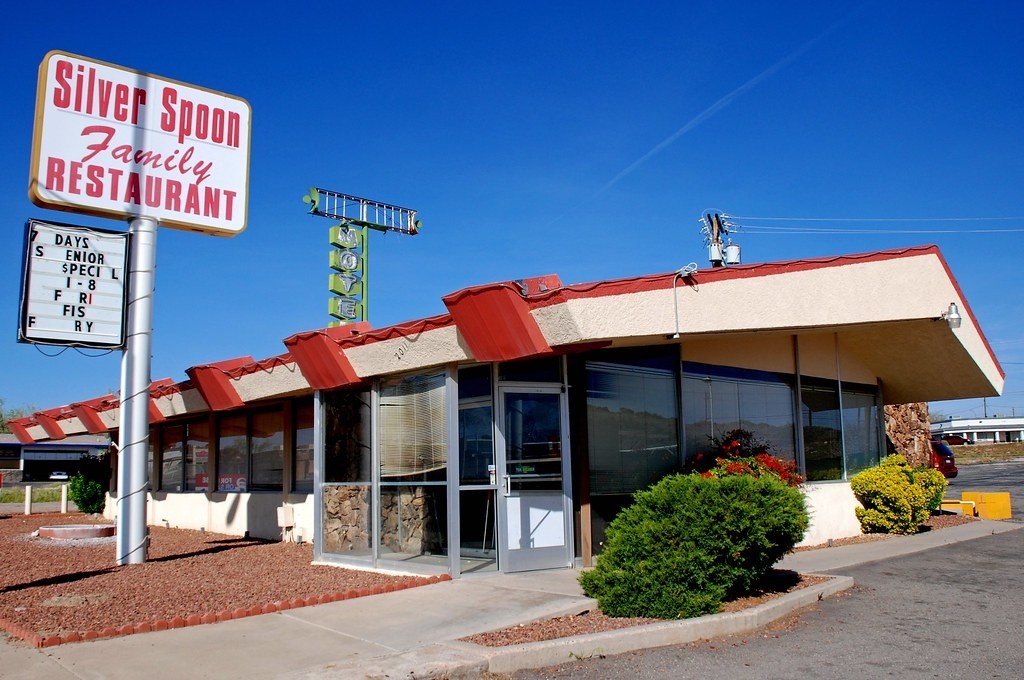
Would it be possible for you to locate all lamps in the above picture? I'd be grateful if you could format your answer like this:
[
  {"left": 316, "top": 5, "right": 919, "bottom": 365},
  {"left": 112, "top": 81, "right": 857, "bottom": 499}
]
[{"left": 935, "top": 302, "right": 960, "bottom": 328}]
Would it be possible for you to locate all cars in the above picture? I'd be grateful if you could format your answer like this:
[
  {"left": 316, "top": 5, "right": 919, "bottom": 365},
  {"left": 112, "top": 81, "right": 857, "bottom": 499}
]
[
  {"left": 49, "top": 471, "right": 68, "bottom": 481},
  {"left": 931, "top": 441, "right": 958, "bottom": 478},
  {"left": 942, "top": 434, "right": 970, "bottom": 446}
]
